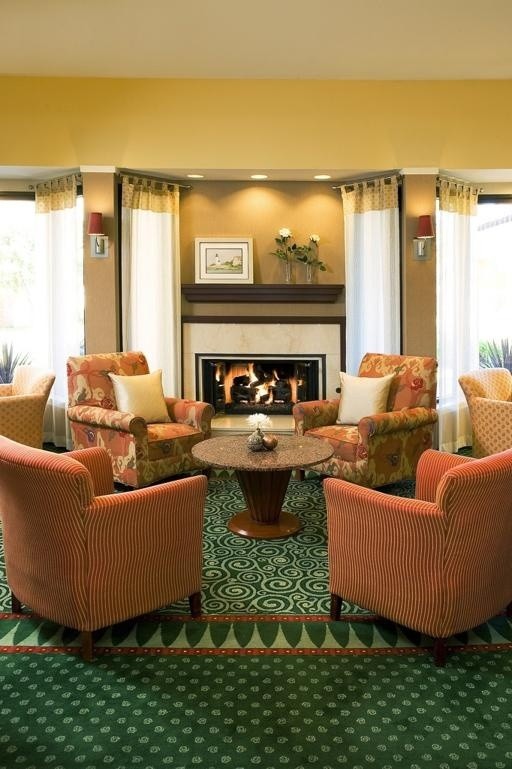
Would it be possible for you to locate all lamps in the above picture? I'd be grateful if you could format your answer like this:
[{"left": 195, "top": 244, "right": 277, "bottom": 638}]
[
  {"left": 87, "top": 211, "right": 109, "bottom": 258},
  {"left": 412, "top": 214, "right": 436, "bottom": 261}
]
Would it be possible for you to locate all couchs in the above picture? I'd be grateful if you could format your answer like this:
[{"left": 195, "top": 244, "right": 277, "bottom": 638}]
[
  {"left": 458, "top": 368, "right": 512, "bottom": 463},
  {"left": 0, "top": 364, "right": 56, "bottom": 455},
  {"left": 323, "top": 441, "right": 512, "bottom": 636},
  {"left": 0, "top": 431, "right": 206, "bottom": 659},
  {"left": 67, "top": 353, "right": 215, "bottom": 489},
  {"left": 291, "top": 354, "right": 440, "bottom": 485}
]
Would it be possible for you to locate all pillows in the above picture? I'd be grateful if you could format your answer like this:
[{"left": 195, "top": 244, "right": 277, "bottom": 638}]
[
  {"left": 336, "top": 370, "right": 397, "bottom": 427},
  {"left": 109, "top": 370, "right": 174, "bottom": 425}
]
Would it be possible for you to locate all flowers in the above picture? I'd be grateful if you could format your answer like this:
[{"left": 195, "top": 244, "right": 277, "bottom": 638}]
[
  {"left": 296, "top": 233, "right": 327, "bottom": 273},
  {"left": 269, "top": 227, "right": 306, "bottom": 264},
  {"left": 245, "top": 412, "right": 275, "bottom": 432}
]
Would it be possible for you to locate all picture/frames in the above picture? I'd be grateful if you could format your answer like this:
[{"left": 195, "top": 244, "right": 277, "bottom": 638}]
[{"left": 194, "top": 237, "right": 255, "bottom": 284}]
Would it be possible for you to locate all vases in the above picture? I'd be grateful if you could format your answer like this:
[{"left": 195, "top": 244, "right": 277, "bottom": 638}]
[
  {"left": 281, "top": 251, "right": 292, "bottom": 283},
  {"left": 305, "top": 261, "right": 315, "bottom": 284},
  {"left": 245, "top": 428, "right": 266, "bottom": 452}
]
